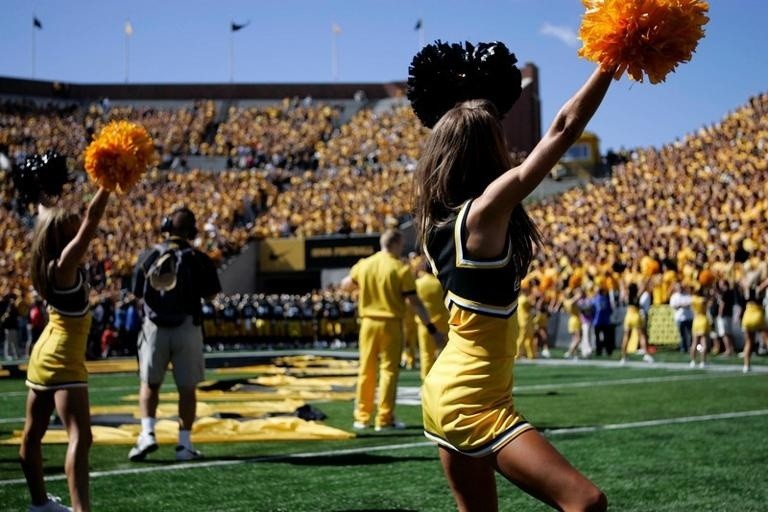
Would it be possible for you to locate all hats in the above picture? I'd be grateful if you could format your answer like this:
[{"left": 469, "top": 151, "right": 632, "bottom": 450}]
[{"left": 148, "top": 249, "right": 179, "bottom": 297}]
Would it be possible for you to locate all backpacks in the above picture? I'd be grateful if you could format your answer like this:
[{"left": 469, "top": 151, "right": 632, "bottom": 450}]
[{"left": 144, "top": 243, "right": 195, "bottom": 330}]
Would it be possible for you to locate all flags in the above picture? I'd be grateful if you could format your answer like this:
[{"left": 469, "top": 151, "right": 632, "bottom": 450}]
[
  {"left": 331, "top": 23, "right": 341, "bottom": 33},
  {"left": 413, "top": 17, "right": 422, "bottom": 31},
  {"left": 231, "top": 20, "right": 251, "bottom": 34},
  {"left": 125, "top": 20, "right": 133, "bottom": 37},
  {"left": 34, "top": 15, "right": 41, "bottom": 28}
]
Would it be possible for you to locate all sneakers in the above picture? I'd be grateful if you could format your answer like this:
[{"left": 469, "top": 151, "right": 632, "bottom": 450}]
[
  {"left": 29, "top": 492, "right": 73, "bottom": 512},
  {"left": 374, "top": 421, "right": 405, "bottom": 431},
  {"left": 542, "top": 348, "right": 766, "bottom": 375},
  {"left": 353, "top": 421, "right": 370, "bottom": 429},
  {"left": 175, "top": 446, "right": 201, "bottom": 460},
  {"left": 128, "top": 432, "right": 159, "bottom": 461}
]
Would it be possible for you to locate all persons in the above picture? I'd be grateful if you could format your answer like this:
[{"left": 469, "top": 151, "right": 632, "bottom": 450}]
[
  {"left": 517, "top": 90, "right": 768, "bottom": 361},
  {"left": 127, "top": 206, "right": 221, "bottom": 462},
  {"left": 409, "top": 62, "right": 617, "bottom": 512},
  {"left": 1, "top": 91, "right": 434, "bottom": 353},
  {"left": 18, "top": 184, "right": 108, "bottom": 512},
  {"left": 338, "top": 228, "right": 448, "bottom": 433},
  {"left": 413, "top": 260, "right": 449, "bottom": 384}
]
